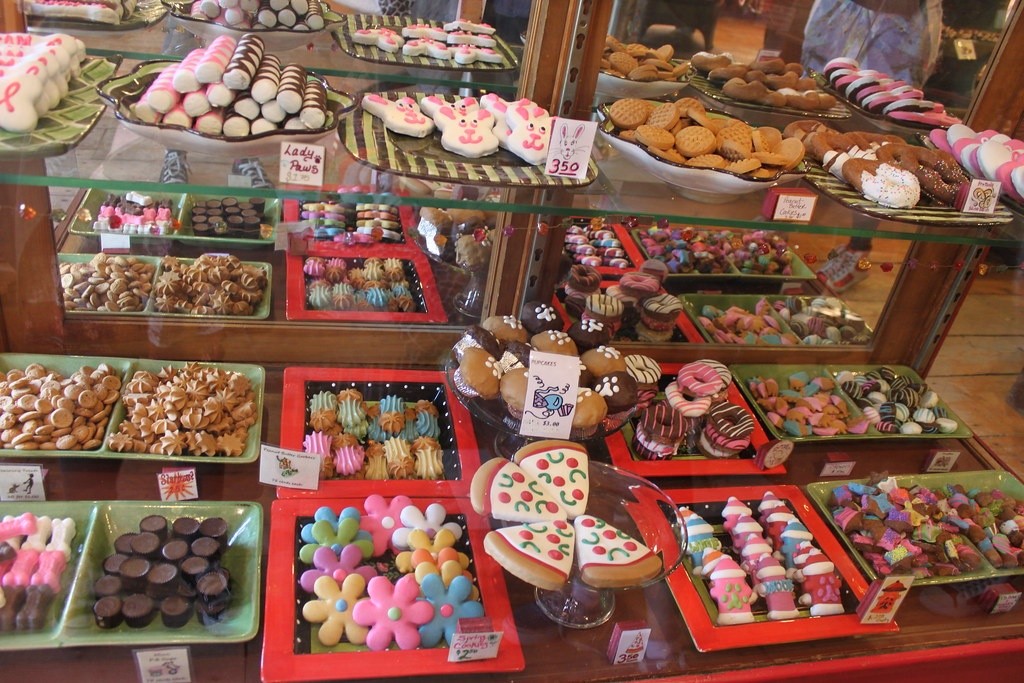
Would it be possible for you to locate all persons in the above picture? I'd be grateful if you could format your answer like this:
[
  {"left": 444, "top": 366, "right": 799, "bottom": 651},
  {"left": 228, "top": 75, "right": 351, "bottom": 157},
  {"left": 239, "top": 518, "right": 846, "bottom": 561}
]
[{"left": 760, "top": 0, "right": 943, "bottom": 293}]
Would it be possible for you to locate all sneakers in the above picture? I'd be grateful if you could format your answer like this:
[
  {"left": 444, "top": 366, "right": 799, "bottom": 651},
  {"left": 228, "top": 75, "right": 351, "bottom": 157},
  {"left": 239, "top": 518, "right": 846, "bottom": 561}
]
[{"left": 818, "top": 245, "right": 871, "bottom": 294}]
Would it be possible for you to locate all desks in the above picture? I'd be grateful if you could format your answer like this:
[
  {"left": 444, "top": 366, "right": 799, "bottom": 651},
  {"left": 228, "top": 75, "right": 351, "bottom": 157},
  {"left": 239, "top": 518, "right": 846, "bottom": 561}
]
[{"left": 0, "top": 362, "right": 1023, "bottom": 683}]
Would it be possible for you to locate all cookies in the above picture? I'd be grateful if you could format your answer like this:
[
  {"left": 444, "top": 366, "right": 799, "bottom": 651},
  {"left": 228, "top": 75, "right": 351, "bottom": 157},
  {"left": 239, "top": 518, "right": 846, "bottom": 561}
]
[
  {"left": 601, "top": 34, "right": 688, "bottom": 81},
  {"left": 59, "top": 251, "right": 416, "bottom": 317},
  {"left": 0, "top": 361, "right": 257, "bottom": 456},
  {"left": 823, "top": 57, "right": 962, "bottom": 127},
  {"left": 925, "top": 124, "right": 1024, "bottom": 206},
  {"left": 609, "top": 98, "right": 804, "bottom": 177},
  {"left": 698, "top": 296, "right": 959, "bottom": 437},
  {"left": 829, "top": 471, "right": 1024, "bottom": 579},
  {"left": 303, "top": 389, "right": 443, "bottom": 481},
  {"left": 301, "top": 203, "right": 400, "bottom": 244}
]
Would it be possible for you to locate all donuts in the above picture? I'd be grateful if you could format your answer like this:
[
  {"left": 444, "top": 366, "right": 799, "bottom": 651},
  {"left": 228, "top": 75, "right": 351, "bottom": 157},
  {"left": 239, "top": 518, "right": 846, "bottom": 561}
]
[
  {"left": 691, "top": 51, "right": 835, "bottom": 111},
  {"left": 784, "top": 119, "right": 968, "bottom": 208},
  {"left": 563, "top": 265, "right": 753, "bottom": 459}
]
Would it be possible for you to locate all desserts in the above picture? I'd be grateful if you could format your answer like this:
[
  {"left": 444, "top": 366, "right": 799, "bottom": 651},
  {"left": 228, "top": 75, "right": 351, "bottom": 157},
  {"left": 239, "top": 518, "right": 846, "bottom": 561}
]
[
  {"left": 675, "top": 490, "right": 845, "bottom": 624},
  {"left": 93, "top": 515, "right": 230, "bottom": 630},
  {"left": 93, "top": 191, "right": 182, "bottom": 235},
  {"left": 400, "top": 178, "right": 638, "bottom": 438},
  {"left": 299, "top": 494, "right": 484, "bottom": 651},
  {"left": 192, "top": 197, "right": 265, "bottom": 240},
  {"left": 0, "top": 0, "right": 552, "bottom": 166},
  {"left": 566, "top": 221, "right": 795, "bottom": 275},
  {"left": 469, "top": 439, "right": 662, "bottom": 591},
  {"left": 0, "top": 512, "right": 77, "bottom": 630}
]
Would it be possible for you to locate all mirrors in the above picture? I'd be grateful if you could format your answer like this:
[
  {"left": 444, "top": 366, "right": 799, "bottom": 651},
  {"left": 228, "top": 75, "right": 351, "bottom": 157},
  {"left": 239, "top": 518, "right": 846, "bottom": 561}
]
[
  {"left": 537, "top": 0, "right": 1016, "bottom": 359},
  {"left": 18, "top": 0, "right": 543, "bottom": 328}
]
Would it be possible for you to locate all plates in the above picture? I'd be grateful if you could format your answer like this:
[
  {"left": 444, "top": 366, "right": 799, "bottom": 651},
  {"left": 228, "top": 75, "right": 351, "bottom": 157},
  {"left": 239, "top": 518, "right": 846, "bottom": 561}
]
[
  {"left": 913, "top": 131, "right": 1023, "bottom": 217},
  {"left": 806, "top": 471, "right": 1024, "bottom": 586},
  {"left": 500, "top": 460, "right": 687, "bottom": 629},
  {"left": 732, "top": 363, "right": 976, "bottom": 442},
  {"left": 0, "top": 353, "right": 265, "bottom": 464},
  {"left": 444, "top": 339, "right": 637, "bottom": 458},
  {"left": 680, "top": 293, "right": 872, "bottom": 347},
  {"left": 518, "top": 30, "right": 697, "bottom": 99},
  {"left": 807, "top": 66, "right": 956, "bottom": 135},
  {"left": 596, "top": 98, "right": 812, "bottom": 195},
  {"left": 332, "top": 14, "right": 520, "bottom": 74},
  {"left": 798, "top": 153, "right": 1013, "bottom": 226},
  {"left": 671, "top": 56, "right": 852, "bottom": 119},
  {"left": 25, "top": 0, "right": 168, "bottom": 36},
  {"left": 632, "top": 227, "right": 816, "bottom": 279},
  {"left": 96, "top": 60, "right": 359, "bottom": 154},
  {"left": 0, "top": 500, "right": 261, "bottom": 652},
  {"left": 163, "top": 1, "right": 347, "bottom": 50},
  {"left": 56, "top": 253, "right": 273, "bottom": 320},
  {"left": 338, "top": 83, "right": 599, "bottom": 188},
  {"left": 1, "top": 54, "right": 123, "bottom": 162},
  {"left": 405, "top": 206, "right": 492, "bottom": 319},
  {"left": 69, "top": 186, "right": 282, "bottom": 250}
]
